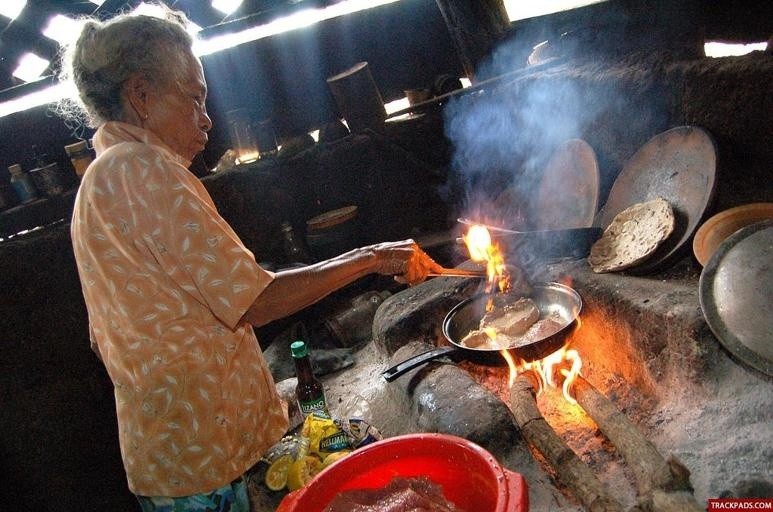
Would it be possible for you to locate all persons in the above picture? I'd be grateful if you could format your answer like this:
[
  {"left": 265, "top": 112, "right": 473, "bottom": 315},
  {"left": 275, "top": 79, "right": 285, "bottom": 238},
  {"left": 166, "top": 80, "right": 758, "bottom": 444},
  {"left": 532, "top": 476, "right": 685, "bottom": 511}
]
[{"left": 60, "top": 15, "right": 445, "bottom": 512}]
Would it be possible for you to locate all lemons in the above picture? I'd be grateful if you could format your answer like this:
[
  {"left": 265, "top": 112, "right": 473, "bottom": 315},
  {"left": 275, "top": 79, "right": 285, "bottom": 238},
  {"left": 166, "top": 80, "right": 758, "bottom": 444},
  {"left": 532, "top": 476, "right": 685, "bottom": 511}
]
[
  {"left": 287, "top": 456, "right": 323, "bottom": 495},
  {"left": 265, "top": 454, "right": 295, "bottom": 491}
]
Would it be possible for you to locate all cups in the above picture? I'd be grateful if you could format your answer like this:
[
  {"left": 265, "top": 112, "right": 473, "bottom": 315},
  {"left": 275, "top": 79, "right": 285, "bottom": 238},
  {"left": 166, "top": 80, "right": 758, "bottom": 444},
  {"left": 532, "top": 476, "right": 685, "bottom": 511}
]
[{"left": 31, "top": 162, "right": 65, "bottom": 200}]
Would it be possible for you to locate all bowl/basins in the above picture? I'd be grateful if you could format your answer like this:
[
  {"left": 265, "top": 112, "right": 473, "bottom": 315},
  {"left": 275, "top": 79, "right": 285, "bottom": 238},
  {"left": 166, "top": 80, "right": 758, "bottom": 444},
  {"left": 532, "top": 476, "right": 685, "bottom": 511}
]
[{"left": 274, "top": 433, "right": 529, "bottom": 512}]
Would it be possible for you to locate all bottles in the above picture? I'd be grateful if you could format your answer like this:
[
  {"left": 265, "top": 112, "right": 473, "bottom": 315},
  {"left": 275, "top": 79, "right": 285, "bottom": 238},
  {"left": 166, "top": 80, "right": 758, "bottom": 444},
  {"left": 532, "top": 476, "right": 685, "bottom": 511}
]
[
  {"left": 251, "top": 116, "right": 279, "bottom": 161},
  {"left": 8, "top": 163, "right": 38, "bottom": 204},
  {"left": 289, "top": 340, "right": 330, "bottom": 418},
  {"left": 279, "top": 220, "right": 312, "bottom": 268},
  {"left": 226, "top": 106, "right": 259, "bottom": 164}
]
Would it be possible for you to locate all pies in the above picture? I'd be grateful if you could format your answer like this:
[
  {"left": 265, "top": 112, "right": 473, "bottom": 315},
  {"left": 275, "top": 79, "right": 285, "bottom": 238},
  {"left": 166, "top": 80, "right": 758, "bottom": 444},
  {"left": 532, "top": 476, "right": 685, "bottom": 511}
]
[{"left": 587, "top": 199, "right": 676, "bottom": 272}]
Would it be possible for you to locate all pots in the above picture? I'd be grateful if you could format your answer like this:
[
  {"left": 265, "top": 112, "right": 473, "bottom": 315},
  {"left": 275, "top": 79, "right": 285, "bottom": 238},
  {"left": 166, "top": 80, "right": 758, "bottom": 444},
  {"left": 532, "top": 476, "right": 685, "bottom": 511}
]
[
  {"left": 380, "top": 282, "right": 584, "bottom": 383},
  {"left": 456, "top": 225, "right": 604, "bottom": 261}
]
